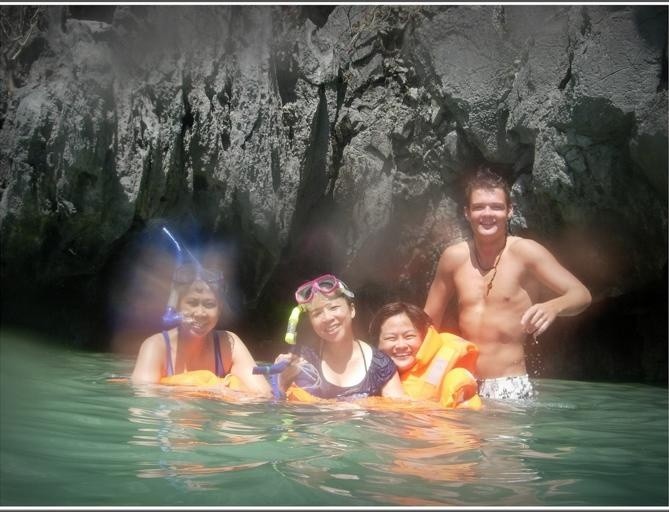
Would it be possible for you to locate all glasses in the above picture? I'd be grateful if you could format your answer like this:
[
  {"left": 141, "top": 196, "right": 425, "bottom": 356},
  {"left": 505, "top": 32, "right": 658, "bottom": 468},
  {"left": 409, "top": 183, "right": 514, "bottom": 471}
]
[
  {"left": 169, "top": 265, "right": 223, "bottom": 283},
  {"left": 295, "top": 274, "right": 354, "bottom": 304}
]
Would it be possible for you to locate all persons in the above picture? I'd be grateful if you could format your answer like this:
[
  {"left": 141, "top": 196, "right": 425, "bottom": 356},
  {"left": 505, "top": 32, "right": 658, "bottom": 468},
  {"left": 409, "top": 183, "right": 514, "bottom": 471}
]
[
  {"left": 423, "top": 173, "right": 592, "bottom": 400},
  {"left": 368, "top": 301, "right": 482, "bottom": 411},
  {"left": 130, "top": 266, "right": 273, "bottom": 398},
  {"left": 274, "top": 273, "right": 404, "bottom": 409}
]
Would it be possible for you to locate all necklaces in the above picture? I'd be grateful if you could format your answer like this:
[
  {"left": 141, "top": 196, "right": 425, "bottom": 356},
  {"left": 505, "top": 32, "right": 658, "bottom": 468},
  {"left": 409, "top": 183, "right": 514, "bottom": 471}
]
[{"left": 474, "top": 235, "right": 507, "bottom": 296}]
